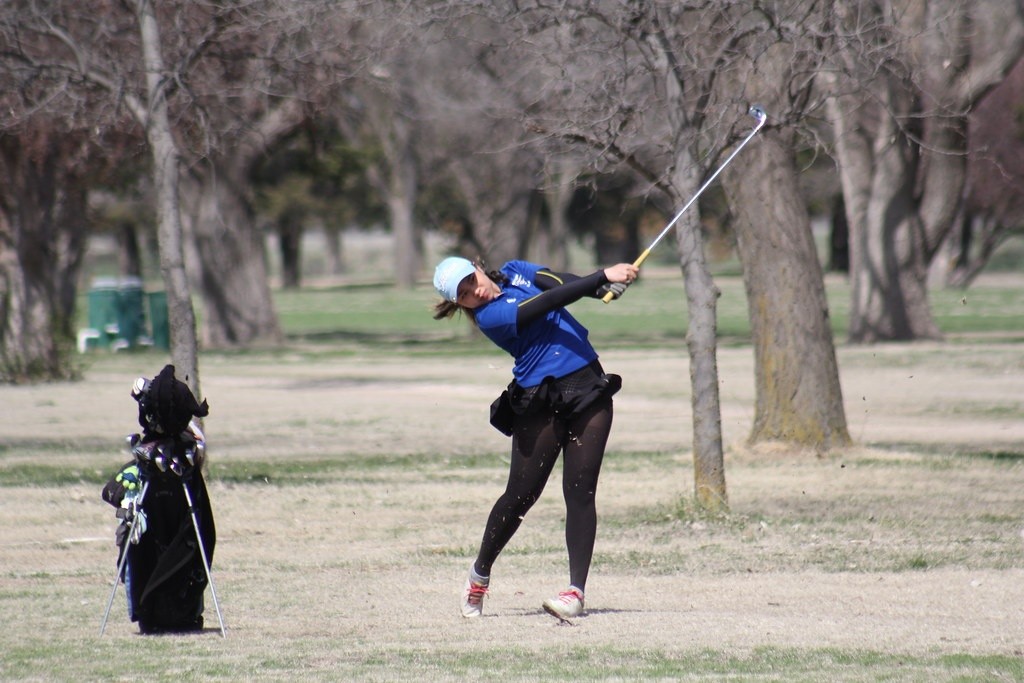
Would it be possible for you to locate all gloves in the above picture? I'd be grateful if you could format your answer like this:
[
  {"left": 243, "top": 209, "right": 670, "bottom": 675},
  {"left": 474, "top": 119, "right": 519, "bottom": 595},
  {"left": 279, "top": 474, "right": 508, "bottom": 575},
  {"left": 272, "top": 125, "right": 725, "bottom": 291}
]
[{"left": 596, "top": 283, "right": 628, "bottom": 299}]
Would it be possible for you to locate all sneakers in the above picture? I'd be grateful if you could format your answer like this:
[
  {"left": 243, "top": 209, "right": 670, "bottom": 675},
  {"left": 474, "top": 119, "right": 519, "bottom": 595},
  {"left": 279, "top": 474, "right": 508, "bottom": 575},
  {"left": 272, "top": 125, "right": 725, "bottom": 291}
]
[
  {"left": 542, "top": 588, "right": 585, "bottom": 621},
  {"left": 460, "top": 578, "right": 489, "bottom": 619}
]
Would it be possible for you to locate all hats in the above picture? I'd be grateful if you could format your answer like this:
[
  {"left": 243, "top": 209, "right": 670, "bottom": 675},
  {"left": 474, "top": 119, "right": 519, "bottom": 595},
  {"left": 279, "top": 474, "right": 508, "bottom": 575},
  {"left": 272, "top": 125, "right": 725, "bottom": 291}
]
[{"left": 433, "top": 256, "right": 476, "bottom": 304}]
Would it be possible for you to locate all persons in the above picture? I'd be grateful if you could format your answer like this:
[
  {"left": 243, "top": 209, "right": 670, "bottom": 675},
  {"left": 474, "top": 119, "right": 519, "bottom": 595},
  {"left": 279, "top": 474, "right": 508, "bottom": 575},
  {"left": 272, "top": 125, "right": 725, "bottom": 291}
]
[{"left": 433, "top": 254, "right": 640, "bottom": 618}]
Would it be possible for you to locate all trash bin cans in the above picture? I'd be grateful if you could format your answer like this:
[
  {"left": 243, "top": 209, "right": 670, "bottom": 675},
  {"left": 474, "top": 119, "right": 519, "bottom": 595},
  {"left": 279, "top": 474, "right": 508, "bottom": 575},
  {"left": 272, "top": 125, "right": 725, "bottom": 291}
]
[
  {"left": 141, "top": 285, "right": 169, "bottom": 352},
  {"left": 89, "top": 276, "right": 146, "bottom": 352}
]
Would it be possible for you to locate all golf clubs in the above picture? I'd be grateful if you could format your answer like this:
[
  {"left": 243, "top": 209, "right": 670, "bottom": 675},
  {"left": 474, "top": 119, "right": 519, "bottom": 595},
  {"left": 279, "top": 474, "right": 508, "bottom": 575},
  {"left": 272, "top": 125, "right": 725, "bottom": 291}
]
[
  {"left": 120, "top": 428, "right": 217, "bottom": 482},
  {"left": 601, "top": 105, "right": 768, "bottom": 303}
]
